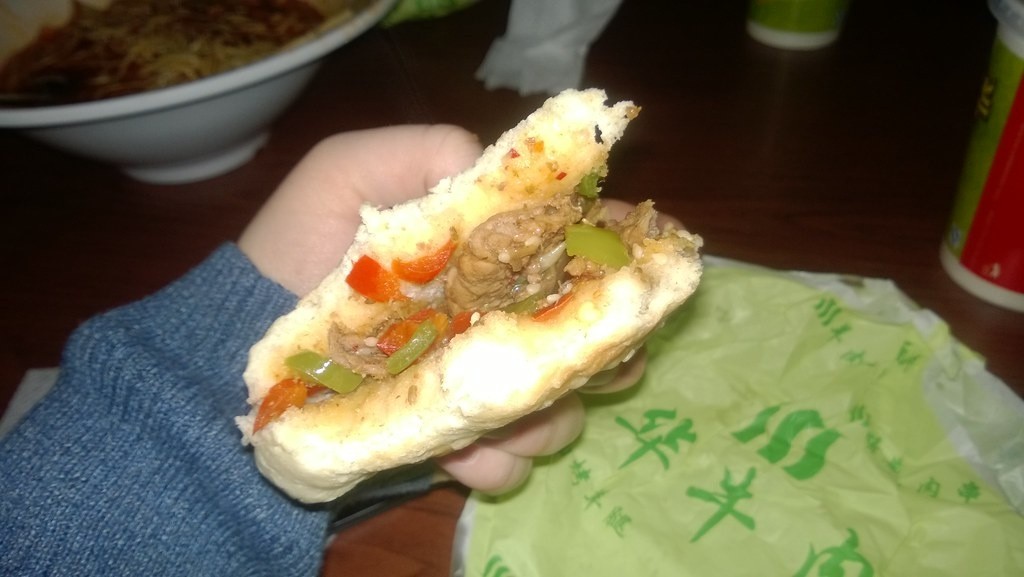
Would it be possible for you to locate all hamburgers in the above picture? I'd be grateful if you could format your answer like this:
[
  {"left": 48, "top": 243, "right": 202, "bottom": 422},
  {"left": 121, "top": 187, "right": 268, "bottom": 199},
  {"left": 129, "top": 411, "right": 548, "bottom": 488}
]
[{"left": 234, "top": 87, "right": 703, "bottom": 504}]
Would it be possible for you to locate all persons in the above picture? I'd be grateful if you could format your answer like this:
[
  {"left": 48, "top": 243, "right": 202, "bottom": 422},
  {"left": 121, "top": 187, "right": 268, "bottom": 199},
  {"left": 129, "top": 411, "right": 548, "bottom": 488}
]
[{"left": 0, "top": 123, "right": 704, "bottom": 576}]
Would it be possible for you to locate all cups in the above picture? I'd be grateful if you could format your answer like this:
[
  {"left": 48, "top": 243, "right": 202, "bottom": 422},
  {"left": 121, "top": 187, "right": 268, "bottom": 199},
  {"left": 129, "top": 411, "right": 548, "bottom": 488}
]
[
  {"left": 942, "top": 1, "right": 1024, "bottom": 310},
  {"left": 745, "top": 0, "right": 848, "bottom": 49}
]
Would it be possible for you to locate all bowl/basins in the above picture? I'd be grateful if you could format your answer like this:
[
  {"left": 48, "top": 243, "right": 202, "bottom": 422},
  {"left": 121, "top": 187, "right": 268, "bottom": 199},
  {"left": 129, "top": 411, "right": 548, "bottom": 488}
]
[{"left": 0, "top": 0, "right": 398, "bottom": 184}]
